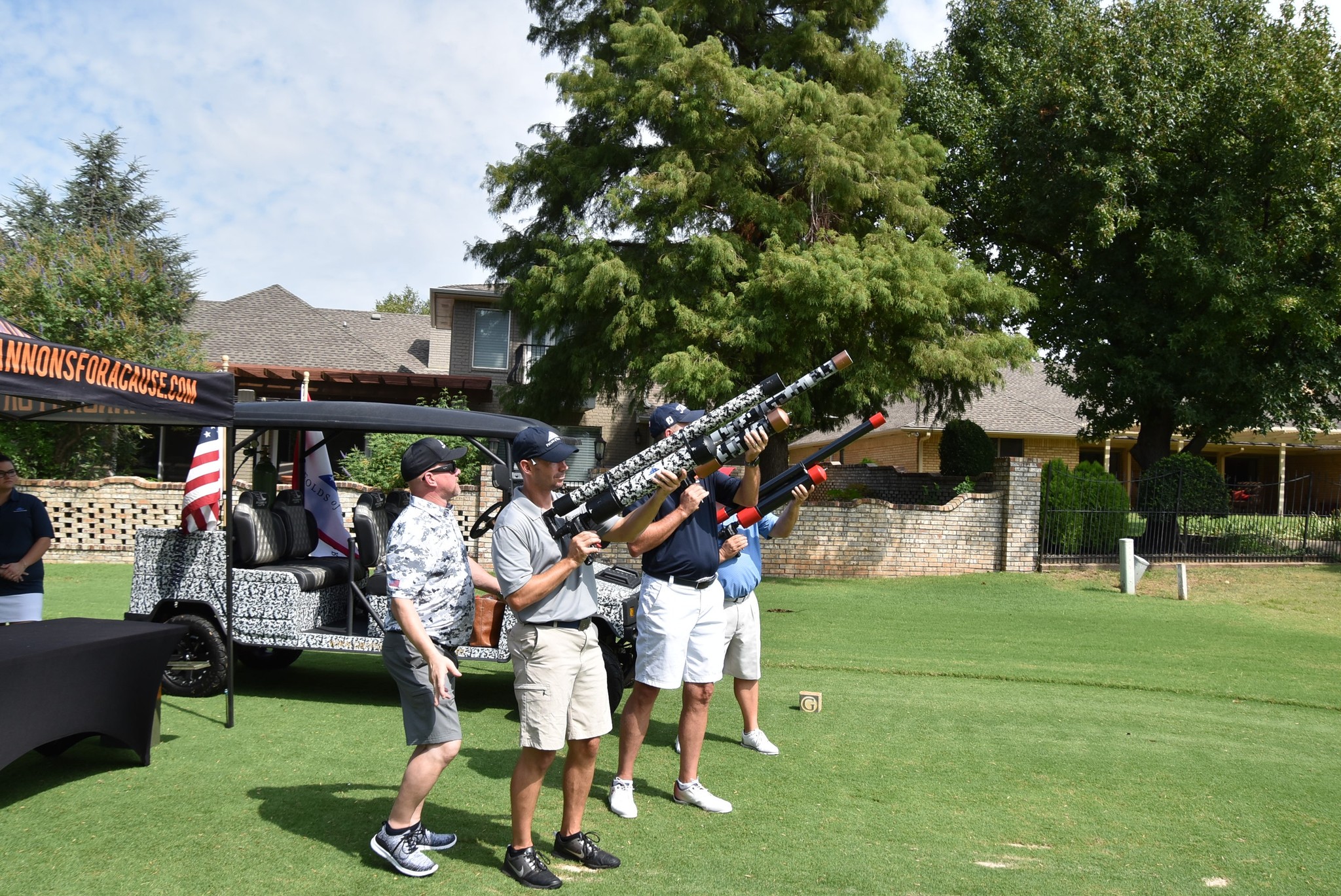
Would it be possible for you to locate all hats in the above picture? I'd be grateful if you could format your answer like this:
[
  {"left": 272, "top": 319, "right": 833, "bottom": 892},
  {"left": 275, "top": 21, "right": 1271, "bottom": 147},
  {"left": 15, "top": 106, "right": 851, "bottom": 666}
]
[
  {"left": 400, "top": 438, "right": 468, "bottom": 482},
  {"left": 511, "top": 427, "right": 580, "bottom": 463},
  {"left": 718, "top": 467, "right": 736, "bottom": 476},
  {"left": 649, "top": 403, "right": 705, "bottom": 438}
]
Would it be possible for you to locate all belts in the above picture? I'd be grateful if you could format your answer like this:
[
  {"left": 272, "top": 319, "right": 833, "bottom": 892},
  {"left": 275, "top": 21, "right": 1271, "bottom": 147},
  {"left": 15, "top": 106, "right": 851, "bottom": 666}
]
[
  {"left": 386, "top": 630, "right": 459, "bottom": 653},
  {"left": 641, "top": 566, "right": 717, "bottom": 589},
  {"left": 524, "top": 616, "right": 592, "bottom": 631},
  {"left": 724, "top": 595, "right": 748, "bottom": 603}
]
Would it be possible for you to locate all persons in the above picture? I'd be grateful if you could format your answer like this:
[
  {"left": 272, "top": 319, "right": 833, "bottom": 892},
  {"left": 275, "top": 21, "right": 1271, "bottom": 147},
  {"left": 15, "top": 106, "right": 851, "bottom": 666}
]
[
  {"left": 369, "top": 437, "right": 504, "bottom": 878},
  {"left": 606, "top": 403, "right": 768, "bottom": 820},
  {"left": 0, "top": 453, "right": 54, "bottom": 622},
  {"left": 493, "top": 425, "right": 686, "bottom": 890},
  {"left": 674, "top": 465, "right": 816, "bottom": 755}
]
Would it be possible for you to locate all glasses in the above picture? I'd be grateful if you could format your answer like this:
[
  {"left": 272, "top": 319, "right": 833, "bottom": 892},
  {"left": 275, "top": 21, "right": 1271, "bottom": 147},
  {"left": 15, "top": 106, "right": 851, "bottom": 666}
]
[
  {"left": 422, "top": 461, "right": 456, "bottom": 480},
  {"left": 0, "top": 469, "right": 17, "bottom": 478}
]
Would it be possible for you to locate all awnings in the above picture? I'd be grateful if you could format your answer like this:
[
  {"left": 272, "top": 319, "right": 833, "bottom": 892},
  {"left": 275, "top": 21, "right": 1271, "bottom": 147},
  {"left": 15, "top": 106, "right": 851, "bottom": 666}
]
[{"left": 1, "top": 318, "right": 236, "bottom": 731}]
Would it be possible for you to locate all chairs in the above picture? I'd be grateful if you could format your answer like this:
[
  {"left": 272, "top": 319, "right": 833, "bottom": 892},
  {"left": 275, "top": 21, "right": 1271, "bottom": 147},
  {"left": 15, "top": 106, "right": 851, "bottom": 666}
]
[
  {"left": 355, "top": 493, "right": 387, "bottom": 639},
  {"left": 232, "top": 493, "right": 345, "bottom": 593},
  {"left": 386, "top": 489, "right": 412, "bottom": 528},
  {"left": 273, "top": 487, "right": 367, "bottom": 582}
]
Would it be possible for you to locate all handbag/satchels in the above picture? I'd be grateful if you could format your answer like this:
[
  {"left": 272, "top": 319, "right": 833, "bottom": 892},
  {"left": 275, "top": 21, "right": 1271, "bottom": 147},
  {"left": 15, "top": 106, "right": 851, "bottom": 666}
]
[{"left": 470, "top": 593, "right": 506, "bottom": 647}]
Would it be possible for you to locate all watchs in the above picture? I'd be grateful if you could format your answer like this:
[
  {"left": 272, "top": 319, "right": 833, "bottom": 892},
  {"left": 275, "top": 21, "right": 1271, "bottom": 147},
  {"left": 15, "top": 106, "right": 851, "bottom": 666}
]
[{"left": 744, "top": 456, "right": 760, "bottom": 467}]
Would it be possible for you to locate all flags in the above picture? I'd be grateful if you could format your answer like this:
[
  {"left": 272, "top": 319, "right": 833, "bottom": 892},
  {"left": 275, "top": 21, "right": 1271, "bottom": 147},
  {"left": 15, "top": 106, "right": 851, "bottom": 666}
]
[
  {"left": 181, "top": 366, "right": 226, "bottom": 536},
  {"left": 291, "top": 377, "right": 367, "bottom": 570}
]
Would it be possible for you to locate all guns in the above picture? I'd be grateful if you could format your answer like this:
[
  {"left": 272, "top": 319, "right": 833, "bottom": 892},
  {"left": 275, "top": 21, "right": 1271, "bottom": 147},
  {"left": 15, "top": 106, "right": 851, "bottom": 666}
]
[
  {"left": 542, "top": 348, "right": 854, "bottom": 568},
  {"left": 678, "top": 404, "right": 793, "bottom": 506},
  {"left": 716, "top": 410, "right": 888, "bottom": 559}
]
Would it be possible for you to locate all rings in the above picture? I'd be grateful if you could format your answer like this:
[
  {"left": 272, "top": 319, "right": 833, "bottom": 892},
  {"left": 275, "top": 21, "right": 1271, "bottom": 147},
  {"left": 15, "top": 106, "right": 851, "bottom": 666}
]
[
  {"left": 11, "top": 575, "right": 13, "bottom": 577},
  {"left": 753, "top": 443, "right": 758, "bottom": 447}
]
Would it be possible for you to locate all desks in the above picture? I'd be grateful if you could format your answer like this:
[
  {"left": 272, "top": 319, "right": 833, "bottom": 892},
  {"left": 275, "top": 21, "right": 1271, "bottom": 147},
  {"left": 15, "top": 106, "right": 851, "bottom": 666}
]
[{"left": 0, "top": 617, "right": 188, "bottom": 766}]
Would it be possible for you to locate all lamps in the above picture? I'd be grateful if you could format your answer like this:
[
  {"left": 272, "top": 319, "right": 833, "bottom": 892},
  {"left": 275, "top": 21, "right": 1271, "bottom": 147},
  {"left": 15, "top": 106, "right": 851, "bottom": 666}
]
[
  {"left": 594, "top": 433, "right": 607, "bottom": 467},
  {"left": 634, "top": 428, "right": 642, "bottom": 444},
  {"left": 487, "top": 438, "right": 500, "bottom": 463}
]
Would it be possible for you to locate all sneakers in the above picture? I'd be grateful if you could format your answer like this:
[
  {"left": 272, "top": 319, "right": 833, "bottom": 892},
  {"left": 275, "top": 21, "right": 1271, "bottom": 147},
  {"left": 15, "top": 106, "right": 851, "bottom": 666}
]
[
  {"left": 370, "top": 821, "right": 439, "bottom": 877},
  {"left": 551, "top": 831, "right": 621, "bottom": 870},
  {"left": 675, "top": 735, "right": 681, "bottom": 753},
  {"left": 413, "top": 822, "right": 457, "bottom": 851},
  {"left": 608, "top": 777, "right": 638, "bottom": 818},
  {"left": 673, "top": 776, "right": 733, "bottom": 813},
  {"left": 740, "top": 729, "right": 779, "bottom": 755},
  {"left": 502, "top": 845, "right": 562, "bottom": 889}
]
